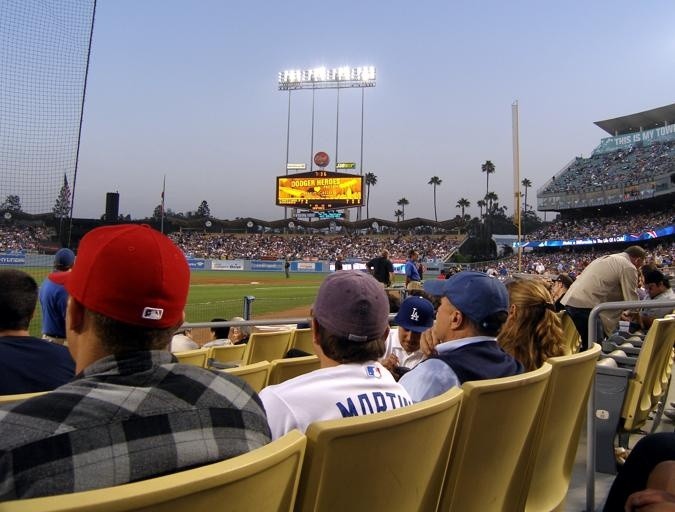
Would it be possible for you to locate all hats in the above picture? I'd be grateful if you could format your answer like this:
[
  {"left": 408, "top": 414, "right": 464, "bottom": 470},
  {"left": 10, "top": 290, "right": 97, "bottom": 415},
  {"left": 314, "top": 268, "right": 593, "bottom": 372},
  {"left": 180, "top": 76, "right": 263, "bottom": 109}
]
[
  {"left": 551, "top": 274, "right": 572, "bottom": 287},
  {"left": 424, "top": 271, "right": 509, "bottom": 322},
  {"left": 312, "top": 270, "right": 390, "bottom": 341},
  {"left": 48, "top": 223, "right": 190, "bottom": 329},
  {"left": 394, "top": 296, "right": 435, "bottom": 332},
  {"left": 55, "top": 247, "right": 76, "bottom": 270}
]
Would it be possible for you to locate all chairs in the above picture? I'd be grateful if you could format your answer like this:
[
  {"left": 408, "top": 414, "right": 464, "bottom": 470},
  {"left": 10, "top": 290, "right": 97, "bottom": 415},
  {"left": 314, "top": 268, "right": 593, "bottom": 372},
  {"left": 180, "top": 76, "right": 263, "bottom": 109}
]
[{"left": 1, "top": 309, "right": 674, "bottom": 512}]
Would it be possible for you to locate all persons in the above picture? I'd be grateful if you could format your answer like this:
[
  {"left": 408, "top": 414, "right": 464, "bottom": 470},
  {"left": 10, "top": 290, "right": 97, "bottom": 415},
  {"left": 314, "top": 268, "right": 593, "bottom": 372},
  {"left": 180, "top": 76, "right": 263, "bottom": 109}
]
[{"left": 1, "top": 141, "right": 675, "bottom": 512}]
